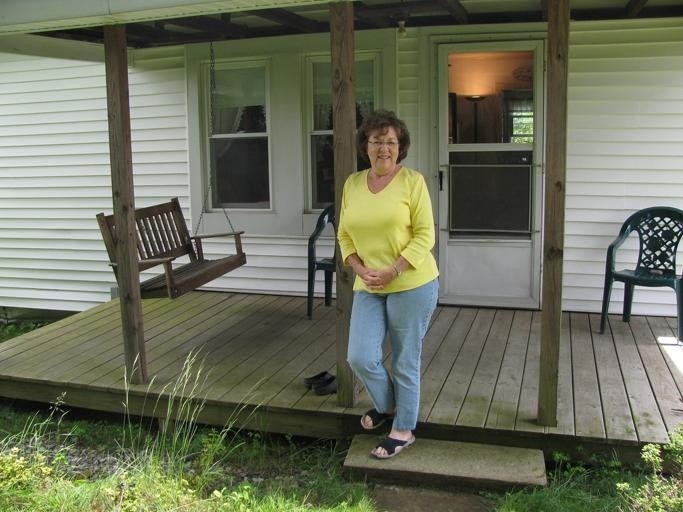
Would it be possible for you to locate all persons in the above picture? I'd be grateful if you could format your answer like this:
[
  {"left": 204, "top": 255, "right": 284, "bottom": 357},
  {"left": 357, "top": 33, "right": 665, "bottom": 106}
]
[{"left": 335, "top": 109, "right": 438, "bottom": 458}]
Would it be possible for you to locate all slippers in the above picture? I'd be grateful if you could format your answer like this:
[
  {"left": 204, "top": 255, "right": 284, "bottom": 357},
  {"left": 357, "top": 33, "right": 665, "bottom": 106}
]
[
  {"left": 360, "top": 408, "right": 395, "bottom": 430},
  {"left": 312, "top": 376, "right": 338, "bottom": 395},
  {"left": 304, "top": 371, "right": 333, "bottom": 388},
  {"left": 370, "top": 434, "right": 416, "bottom": 459}
]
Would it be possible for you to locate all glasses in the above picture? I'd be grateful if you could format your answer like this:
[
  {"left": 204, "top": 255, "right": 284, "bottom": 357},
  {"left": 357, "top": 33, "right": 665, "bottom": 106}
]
[{"left": 368, "top": 139, "right": 396, "bottom": 147}]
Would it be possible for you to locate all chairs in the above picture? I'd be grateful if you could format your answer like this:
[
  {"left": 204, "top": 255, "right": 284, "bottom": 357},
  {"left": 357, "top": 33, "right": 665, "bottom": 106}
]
[
  {"left": 306, "top": 203, "right": 336, "bottom": 320},
  {"left": 599, "top": 206, "right": 683, "bottom": 346}
]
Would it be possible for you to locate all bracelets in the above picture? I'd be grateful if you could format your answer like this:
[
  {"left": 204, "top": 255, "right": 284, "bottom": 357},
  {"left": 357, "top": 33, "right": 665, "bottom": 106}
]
[{"left": 392, "top": 264, "right": 401, "bottom": 276}]
